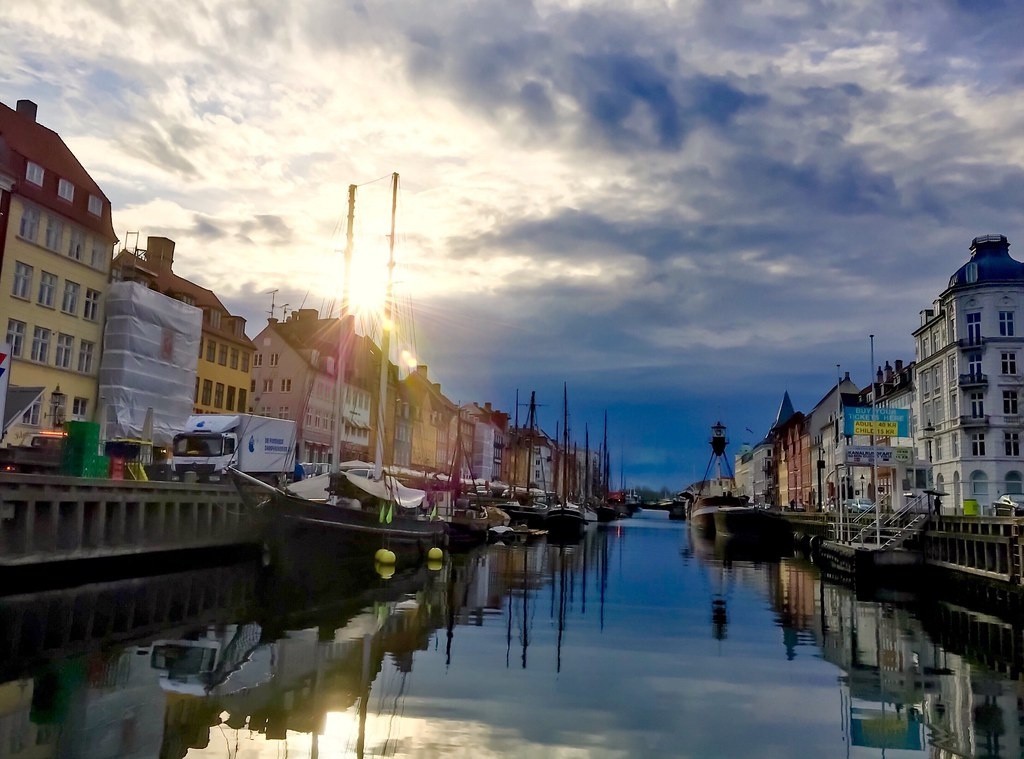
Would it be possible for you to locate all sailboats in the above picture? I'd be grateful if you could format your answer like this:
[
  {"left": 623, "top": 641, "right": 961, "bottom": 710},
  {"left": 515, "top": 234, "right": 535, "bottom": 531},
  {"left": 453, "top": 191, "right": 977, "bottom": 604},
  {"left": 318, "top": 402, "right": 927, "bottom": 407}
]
[
  {"left": 228, "top": 171, "right": 452, "bottom": 562},
  {"left": 422, "top": 406, "right": 512, "bottom": 543},
  {"left": 502, "top": 378, "right": 642, "bottom": 544}
]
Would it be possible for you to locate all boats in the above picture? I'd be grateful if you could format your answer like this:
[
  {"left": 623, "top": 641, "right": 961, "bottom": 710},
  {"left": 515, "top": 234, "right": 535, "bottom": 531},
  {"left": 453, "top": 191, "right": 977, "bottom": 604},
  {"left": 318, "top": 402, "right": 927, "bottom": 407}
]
[
  {"left": 688, "top": 421, "right": 762, "bottom": 555},
  {"left": 491, "top": 523, "right": 549, "bottom": 545},
  {"left": 667, "top": 492, "right": 696, "bottom": 521}
]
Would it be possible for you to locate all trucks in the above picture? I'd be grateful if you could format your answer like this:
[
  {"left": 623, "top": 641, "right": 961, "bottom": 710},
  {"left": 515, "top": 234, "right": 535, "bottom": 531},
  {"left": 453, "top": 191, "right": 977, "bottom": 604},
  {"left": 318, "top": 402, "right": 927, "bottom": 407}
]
[
  {"left": 148, "top": 622, "right": 280, "bottom": 695},
  {"left": 173, "top": 413, "right": 297, "bottom": 482}
]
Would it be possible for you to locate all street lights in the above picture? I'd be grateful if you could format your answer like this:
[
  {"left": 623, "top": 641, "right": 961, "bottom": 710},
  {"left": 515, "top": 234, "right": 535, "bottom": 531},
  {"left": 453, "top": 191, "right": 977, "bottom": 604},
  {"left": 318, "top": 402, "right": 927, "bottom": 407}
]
[
  {"left": 860, "top": 474, "right": 865, "bottom": 498},
  {"left": 51, "top": 384, "right": 63, "bottom": 428}
]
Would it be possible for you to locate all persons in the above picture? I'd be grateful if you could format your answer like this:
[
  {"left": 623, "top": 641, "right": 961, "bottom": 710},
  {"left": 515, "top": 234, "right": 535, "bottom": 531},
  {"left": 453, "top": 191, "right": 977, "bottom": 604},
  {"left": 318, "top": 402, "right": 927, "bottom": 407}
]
[
  {"left": 933, "top": 497, "right": 942, "bottom": 515},
  {"left": 790, "top": 499, "right": 795, "bottom": 512}
]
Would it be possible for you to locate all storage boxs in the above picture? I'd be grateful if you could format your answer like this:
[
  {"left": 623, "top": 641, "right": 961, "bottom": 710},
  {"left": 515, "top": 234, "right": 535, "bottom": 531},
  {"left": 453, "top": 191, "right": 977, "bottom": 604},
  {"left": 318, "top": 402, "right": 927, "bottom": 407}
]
[{"left": 61, "top": 420, "right": 124, "bottom": 479}]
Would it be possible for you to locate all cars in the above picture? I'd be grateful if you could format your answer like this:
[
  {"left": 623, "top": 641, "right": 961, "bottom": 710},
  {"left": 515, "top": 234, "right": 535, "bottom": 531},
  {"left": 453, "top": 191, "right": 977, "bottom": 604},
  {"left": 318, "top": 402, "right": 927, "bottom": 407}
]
[
  {"left": 820, "top": 497, "right": 854, "bottom": 513},
  {"left": 788, "top": 503, "right": 805, "bottom": 512},
  {"left": 991, "top": 493, "right": 1024, "bottom": 517},
  {"left": 851, "top": 498, "right": 876, "bottom": 514}
]
